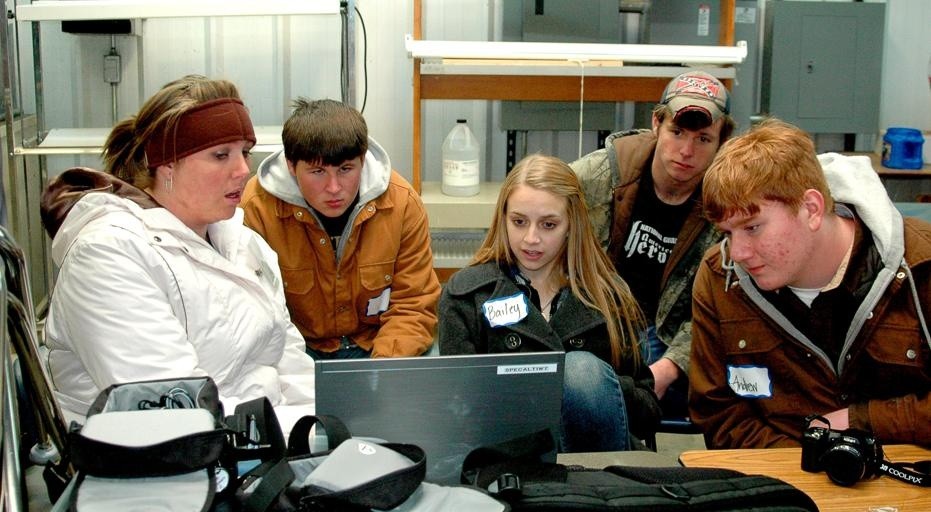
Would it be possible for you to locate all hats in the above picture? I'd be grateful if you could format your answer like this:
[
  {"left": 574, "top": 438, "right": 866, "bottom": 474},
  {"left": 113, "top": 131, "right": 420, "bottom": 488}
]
[{"left": 659, "top": 70, "right": 731, "bottom": 124}]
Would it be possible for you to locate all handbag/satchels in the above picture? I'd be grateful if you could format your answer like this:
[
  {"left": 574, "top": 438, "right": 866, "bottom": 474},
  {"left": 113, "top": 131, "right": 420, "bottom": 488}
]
[
  {"left": 77, "top": 376, "right": 506, "bottom": 512},
  {"left": 461, "top": 429, "right": 819, "bottom": 512}
]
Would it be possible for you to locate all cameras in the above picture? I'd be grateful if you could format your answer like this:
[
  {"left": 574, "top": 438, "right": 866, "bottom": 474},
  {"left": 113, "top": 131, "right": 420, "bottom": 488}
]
[{"left": 799, "top": 425, "right": 882, "bottom": 487}]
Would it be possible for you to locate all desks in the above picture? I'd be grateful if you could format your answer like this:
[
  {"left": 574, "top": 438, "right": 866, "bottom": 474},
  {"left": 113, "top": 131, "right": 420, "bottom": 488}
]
[
  {"left": 678, "top": 444, "right": 931, "bottom": 512},
  {"left": 845, "top": 150, "right": 931, "bottom": 180},
  {"left": 556, "top": 450, "right": 681, "bottom": 470}
]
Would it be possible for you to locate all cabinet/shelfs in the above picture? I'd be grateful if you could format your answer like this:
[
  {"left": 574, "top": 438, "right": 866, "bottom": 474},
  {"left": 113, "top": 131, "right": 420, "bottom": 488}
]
[
  {"left": 1, "top": 1, "right": 351, "bottom": 465},
  {"left": 413, "top": 0, "right": 734, "bottom": 230}
]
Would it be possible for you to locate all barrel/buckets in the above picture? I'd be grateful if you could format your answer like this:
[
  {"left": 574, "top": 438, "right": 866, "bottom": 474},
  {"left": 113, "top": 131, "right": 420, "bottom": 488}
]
[{"left": 440, "top": 117, "right": 482, "bottom": 199}]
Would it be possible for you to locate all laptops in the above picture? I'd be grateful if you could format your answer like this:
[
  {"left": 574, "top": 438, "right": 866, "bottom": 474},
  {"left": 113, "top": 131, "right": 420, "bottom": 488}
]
[{"left": 313, "top": 351, "right": 565, "bottom": 485}]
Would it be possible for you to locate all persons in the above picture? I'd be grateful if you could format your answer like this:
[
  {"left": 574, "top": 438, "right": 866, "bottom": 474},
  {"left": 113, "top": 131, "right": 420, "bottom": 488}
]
[
  {"left": 237, "top": 98, "right": 443, "bottom": 361},
  {"left": 437, "top": 153, "right": 661, "bottom": 449},
  {"left": 687, "top": 119, "right": 931, "bottom": 450},
  {"left": 40, "top": 75, "right": 317, "bottom": 446},
  {"left": 566, "top": 71, "right": 739, "bottom": 414}
]
